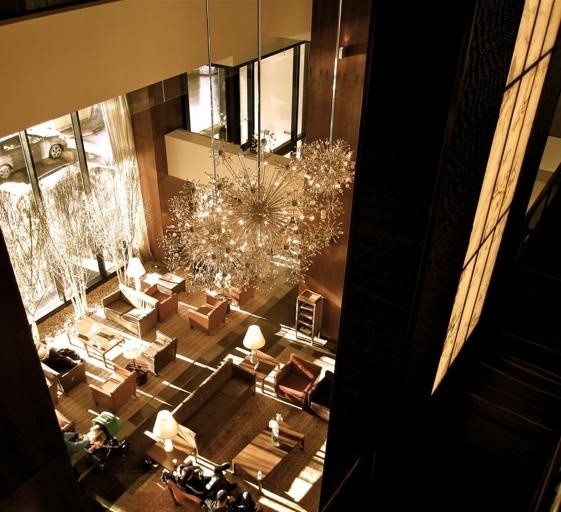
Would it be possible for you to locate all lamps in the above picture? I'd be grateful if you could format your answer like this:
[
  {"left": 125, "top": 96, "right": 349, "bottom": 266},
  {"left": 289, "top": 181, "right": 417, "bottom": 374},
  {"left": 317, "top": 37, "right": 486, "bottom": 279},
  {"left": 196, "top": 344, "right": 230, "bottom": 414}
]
[
  {"left": 157, "top": 132, "right": 355, "bottom": 293},
  {"left": 152, "top": 410, "right": 180, "bottom": 452},
  {"left": 243, "top": 325, "right": 266, "bottom": 371},
  {"left": 126, "top": 258, "right": 145, "bottom": 290},
  {"left": 299, "top": 0, "right": 356, "bottom": 196}
]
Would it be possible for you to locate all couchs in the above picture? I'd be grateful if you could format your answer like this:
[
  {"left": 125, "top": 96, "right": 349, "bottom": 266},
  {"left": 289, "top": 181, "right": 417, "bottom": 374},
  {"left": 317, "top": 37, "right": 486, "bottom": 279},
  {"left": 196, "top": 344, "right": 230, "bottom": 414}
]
[
  {"left": 101, "top": 284, "right": 160, "bottom": 340},
  {"left": 89, "top": 365, "right": 136, "bottom": 415},
  {"left": 40, "top": 354, "right": 87, "bottom": 395},
  {"left": 187, "top": 293, "right": 228, "bottom": 336},
  {"left": 171, "top": 358, "right": 256, "bottom": 452},
  {"left": 274, "top": 352, "right": 322, "bottom": 411},
  {"left": 308, "top": 370, "right": 334, "bottom": 422},
  {"left": 229, "top": 273, "right": 258, "bottom": 310},
  {"left": 139, "top": 330, "right": 178, "bottom": 377}
]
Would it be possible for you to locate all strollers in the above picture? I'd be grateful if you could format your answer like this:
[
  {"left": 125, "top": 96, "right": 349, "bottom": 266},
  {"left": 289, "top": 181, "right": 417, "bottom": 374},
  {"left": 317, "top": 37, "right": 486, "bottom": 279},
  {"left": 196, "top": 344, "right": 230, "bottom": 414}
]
[{"left": 82, "top": 411, "right": 127, "bottom": 470}]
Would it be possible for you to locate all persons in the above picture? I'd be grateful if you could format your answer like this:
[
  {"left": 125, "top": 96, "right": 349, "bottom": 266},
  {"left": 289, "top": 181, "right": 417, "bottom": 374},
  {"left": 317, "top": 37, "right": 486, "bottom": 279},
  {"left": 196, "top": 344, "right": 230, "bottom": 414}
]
[
  {"left": 205, "top": 489, "right": 235, "bottom": 512},
  {"left": 175, "top": 461, "right": 207, "bottom": 492},
  {"left": 61, "top": 429, "right": 89, "bottom": 456}
]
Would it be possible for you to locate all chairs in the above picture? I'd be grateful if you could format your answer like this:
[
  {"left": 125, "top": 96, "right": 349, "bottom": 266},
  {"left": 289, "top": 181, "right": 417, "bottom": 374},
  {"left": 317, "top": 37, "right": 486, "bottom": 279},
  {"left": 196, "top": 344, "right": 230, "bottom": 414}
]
[{"left": 164, "top": 460, "right": 203, "bottom": 512}]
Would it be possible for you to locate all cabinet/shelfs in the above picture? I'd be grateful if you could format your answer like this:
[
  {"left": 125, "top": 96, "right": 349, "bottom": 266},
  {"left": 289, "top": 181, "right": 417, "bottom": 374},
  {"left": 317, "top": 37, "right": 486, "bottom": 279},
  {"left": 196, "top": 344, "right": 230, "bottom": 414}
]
[
  {"left": 239, "top": 349, "right": 280, "bottom": 393},
  {"left": 296, "top": 289, "right": 323, "bottom": 347},
  {"left": 144, "top": 282, "right": 178, "bottom": 322},
  {"left": 146, "top": 440, "right": 197, "bottom": 473}
]
